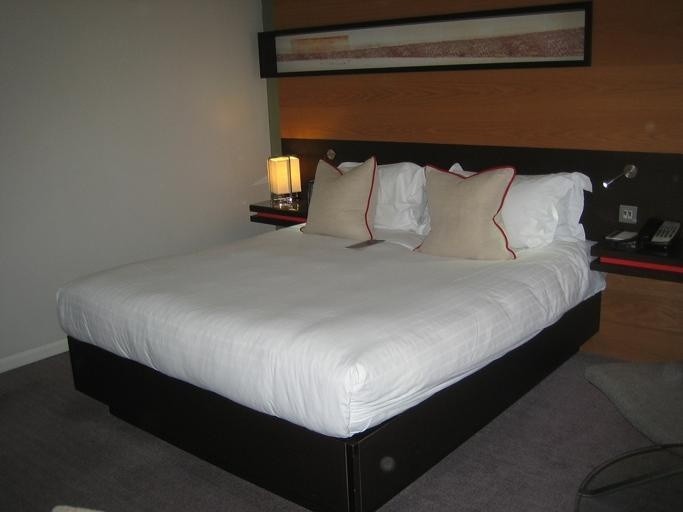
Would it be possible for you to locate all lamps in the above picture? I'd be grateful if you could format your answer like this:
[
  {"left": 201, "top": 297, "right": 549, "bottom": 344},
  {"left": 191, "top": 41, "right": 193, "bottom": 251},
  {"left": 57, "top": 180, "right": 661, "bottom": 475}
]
[{"left": 267, "top": 154, "right": 303, "bottom": 196}]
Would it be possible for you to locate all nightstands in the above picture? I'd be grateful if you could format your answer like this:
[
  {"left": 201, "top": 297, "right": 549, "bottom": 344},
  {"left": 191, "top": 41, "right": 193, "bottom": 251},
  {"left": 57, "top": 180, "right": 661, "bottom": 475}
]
[
  {"left": 580, "top": 242, "right": 683, "bottom": 361},
  {"left": 249, "top": 198, "right": 307, "bottom": 229}
]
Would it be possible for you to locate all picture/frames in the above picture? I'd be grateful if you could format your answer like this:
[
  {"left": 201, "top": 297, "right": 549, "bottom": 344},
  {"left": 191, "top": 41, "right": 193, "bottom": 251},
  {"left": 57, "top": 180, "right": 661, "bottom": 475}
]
[{"left": 256, "top": 0, "right": 593, "bottom": 80}]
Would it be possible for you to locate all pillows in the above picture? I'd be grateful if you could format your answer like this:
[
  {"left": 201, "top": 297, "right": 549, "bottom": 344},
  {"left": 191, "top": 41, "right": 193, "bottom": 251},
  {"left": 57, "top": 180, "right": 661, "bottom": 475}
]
[
  {"left": 553, "top": 172, "right": 593, "bottom": 242},
  {"left": 337, "top": 160, "right": 429, "bottom": 233},
  {"left": 300, "top": 157, "right": 379, "bottom": 241},
  {"left": 414, "top": 164, "right": 517, "bottom": 261},
  {"left": 417, "top": 168, "right": 574, "bottom": 249}
]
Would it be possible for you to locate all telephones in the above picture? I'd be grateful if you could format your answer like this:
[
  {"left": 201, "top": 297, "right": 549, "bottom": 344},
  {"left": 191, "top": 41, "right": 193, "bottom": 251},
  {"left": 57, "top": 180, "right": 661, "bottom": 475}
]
[{"left": 635, "top": 217, "right": 681, "bottom": 257}]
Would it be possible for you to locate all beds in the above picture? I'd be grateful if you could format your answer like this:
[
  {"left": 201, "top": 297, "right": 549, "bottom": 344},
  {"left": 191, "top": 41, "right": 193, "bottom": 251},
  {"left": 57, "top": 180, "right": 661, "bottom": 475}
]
[{"left": 55, "top": 224, "right": 603, "bottom": 511}]
